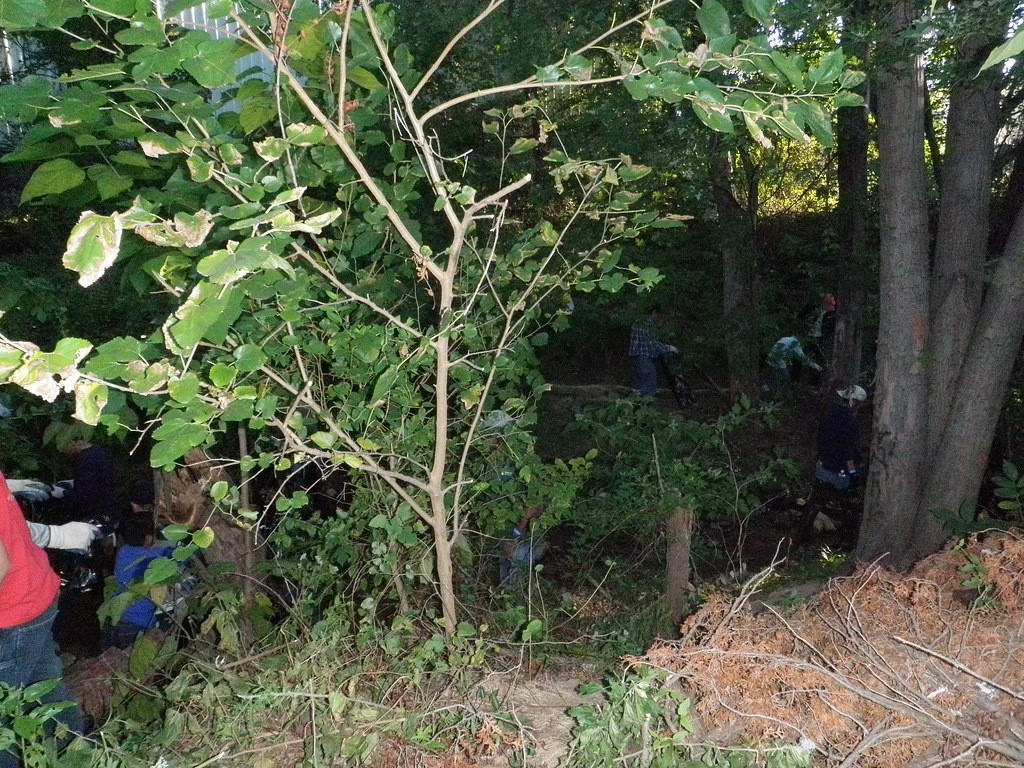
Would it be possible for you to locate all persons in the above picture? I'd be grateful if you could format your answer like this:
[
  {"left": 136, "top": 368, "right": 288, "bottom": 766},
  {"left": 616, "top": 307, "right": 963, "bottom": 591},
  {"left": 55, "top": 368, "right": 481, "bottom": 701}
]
[
  {"left": 0, "top": 408, "right": 206, "bottom": 768},
  {"left": 758, "top": 330, "right": 822, "bottom": 406},
  {"left": 628, "top": 303, "right": 678, "bottom": 400},
  {"left": 811, "top": 384, "right": 870, "bottom": 534}
]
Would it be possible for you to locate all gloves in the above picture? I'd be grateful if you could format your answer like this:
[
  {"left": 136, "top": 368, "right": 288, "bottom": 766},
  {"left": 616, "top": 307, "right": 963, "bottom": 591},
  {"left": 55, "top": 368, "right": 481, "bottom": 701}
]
[
  {"left": 809, "top": 362, "right": 822, "bottom": 371},
  {"left": 51, "top": 484, "right": 66, "bottom": 498},
  {"left": 5, "top": 479, "right": 51, "bottom": 501},
  {"left": 45, "top": 521, "right": 100, "bottom": 551},
  {"left": 663, "top": 344, "right": 679, "bottom": 354},
  {"left": 55, "top": 480, "right": 75, "bottom": 489},
  {"left": 849, "top": 468, "right": 862, "bottom": 487}
]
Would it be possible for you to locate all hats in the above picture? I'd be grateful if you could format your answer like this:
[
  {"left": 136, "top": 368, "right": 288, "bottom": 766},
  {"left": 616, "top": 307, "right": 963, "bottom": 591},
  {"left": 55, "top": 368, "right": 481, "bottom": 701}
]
[
  {"left": 824, "top": 296, "right": 836, "bottom": 311},
  {"left": 476, "top": 409, "right": 512, "bottom": 430},
  {"left": 0, "top": 404, "right": 12, "bottom": 420},
  {"left": 131, "top": 483, "right": 154, "bottom": 504},
  {"left": 837, "top": 385, "right": 866, "bottom": 401}
]
